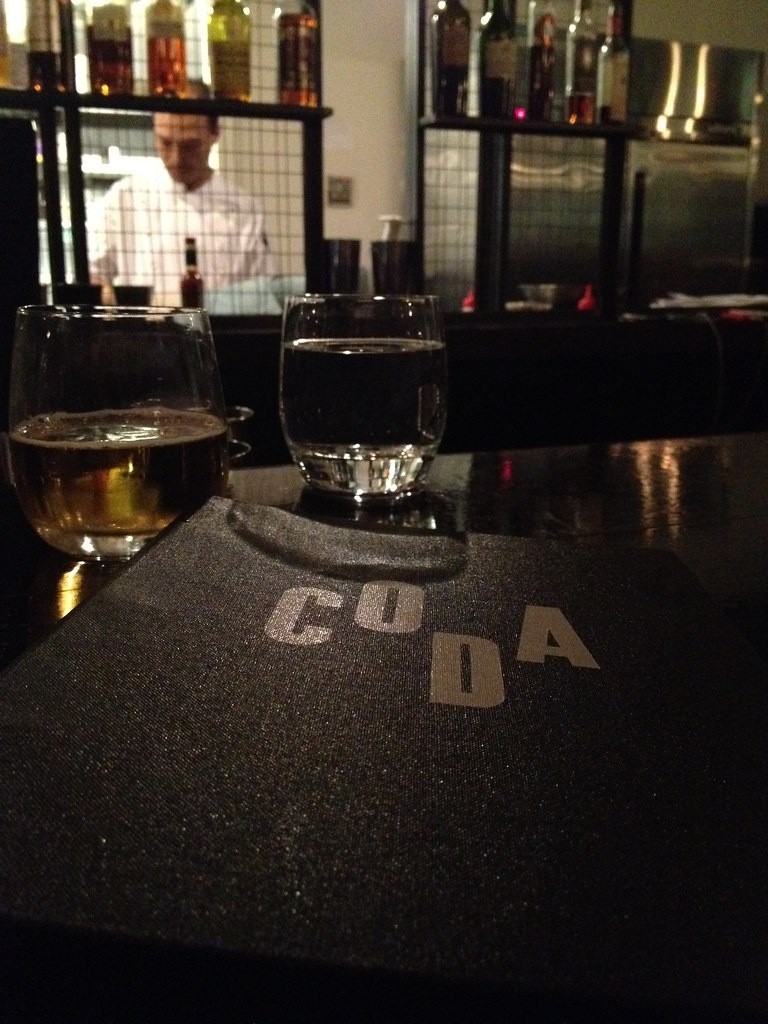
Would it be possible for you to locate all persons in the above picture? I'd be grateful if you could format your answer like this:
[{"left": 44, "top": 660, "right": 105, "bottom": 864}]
[{"left": 61, "top": 77, "right": 282, "bottom": 320}]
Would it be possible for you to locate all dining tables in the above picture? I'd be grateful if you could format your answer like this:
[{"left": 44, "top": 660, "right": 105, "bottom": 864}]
[{"left": 7, "top": 461, "right": 767, "bottom": 1023}]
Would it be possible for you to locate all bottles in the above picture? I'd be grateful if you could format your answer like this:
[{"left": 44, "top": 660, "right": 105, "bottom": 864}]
[
  {"left": 475, "top": 5, "right": 515, "bottom": 114},
  {"left": 178, "top": 236, "right": 206, "bottom": 311},
  {"left": 27, "top": 0, "right": 69, "bottom": 91},
  {"left": 429, "top": 2, "right": 471, "bottom": 120},
  {"left": 208, "top": 1, "right": 251, "bottom": 100},
  {"left": 524, "top": 3, "right": 559, "bottom": 119},
  {"left": 85, "top": 3, "right": 137, "bottom": 98},
  {"left": 147, "top": 2, "right": 186, "bottom": 101},
  {"left": 276, "top": 3, "right": 319, "bottom": 107},
  {"left": 599, "top": 7, "right": 628, "bottom": 129},
  {"left": 566, "top": 1, "right": 592, "bottom": 125}
]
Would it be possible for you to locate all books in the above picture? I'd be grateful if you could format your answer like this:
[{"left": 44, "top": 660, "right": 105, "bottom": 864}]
[{"left": 0, "top": 489, "right": 768, "bottom": 1024}]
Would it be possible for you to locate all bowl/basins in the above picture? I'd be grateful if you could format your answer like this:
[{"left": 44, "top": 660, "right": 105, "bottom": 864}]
[{"left": 517, "top": 283, "right": 566, "bottom": 304}]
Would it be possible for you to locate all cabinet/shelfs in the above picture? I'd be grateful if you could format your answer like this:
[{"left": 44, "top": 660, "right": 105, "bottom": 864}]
[
  {"left": 0, "top": 0, "right": 333, "bottom": 412},
  {"left": 405, "top": 1, "right": 768, "bottom": 415}
]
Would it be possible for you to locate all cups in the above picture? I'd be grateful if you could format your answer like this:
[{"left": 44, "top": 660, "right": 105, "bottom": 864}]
[
  {"left": 281, "top": 288, "right": 442, "bottom": 500},
  {"left": 0, "top": 298, "right": 230, "bottom": 564}
]
